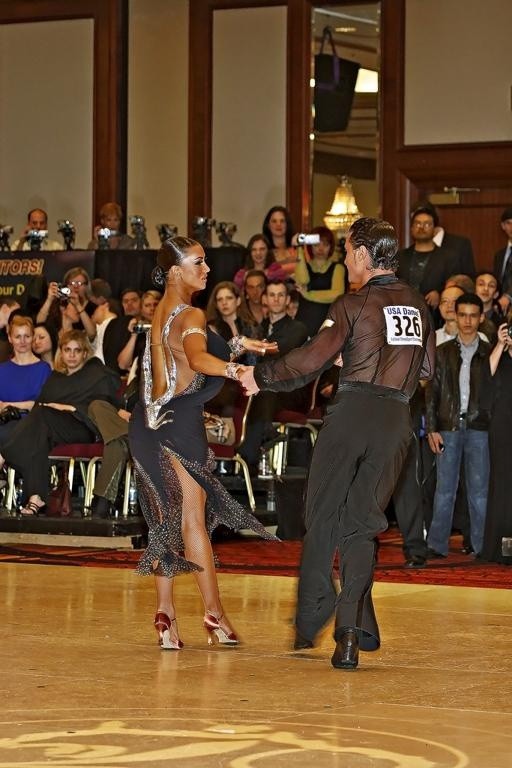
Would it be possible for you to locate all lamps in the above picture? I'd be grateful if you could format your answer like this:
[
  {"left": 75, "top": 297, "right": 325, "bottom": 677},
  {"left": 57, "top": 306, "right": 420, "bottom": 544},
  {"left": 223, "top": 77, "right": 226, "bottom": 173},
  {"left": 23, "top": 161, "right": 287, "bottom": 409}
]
[{"left": 323, "top": 175, "right": 364, "bottom": 240}]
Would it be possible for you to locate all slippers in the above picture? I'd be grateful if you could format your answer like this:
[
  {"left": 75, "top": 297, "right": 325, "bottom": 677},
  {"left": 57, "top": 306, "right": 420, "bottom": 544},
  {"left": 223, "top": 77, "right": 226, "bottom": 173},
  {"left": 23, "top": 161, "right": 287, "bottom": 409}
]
[{"left": 22, "top": 502, "right": 46, "bottom": 516}]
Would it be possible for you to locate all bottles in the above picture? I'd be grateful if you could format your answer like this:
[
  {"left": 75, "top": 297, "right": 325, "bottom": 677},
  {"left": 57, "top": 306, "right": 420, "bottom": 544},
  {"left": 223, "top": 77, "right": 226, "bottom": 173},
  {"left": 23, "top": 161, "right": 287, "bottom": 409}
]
[
  {"left": 265, "top": 479, "right": 277, "bottom": 514},
  {"left": 259, "top": 452, "right": 270, "bottom": 478},
  {"left": 128, "top": 480, "right": 139, "bottom": 515}
]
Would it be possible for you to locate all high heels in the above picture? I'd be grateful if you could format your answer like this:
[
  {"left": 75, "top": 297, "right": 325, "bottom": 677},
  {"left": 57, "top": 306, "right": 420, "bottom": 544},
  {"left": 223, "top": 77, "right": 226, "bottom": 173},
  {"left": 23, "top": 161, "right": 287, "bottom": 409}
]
[
  {"left": 153, "top": 612, "right": 183, "bottom": 649},
  {"left": 202, "top": 613, "right": 240, "bottom": 644},
  {"left": 331, "top": 630, "right": 359, "bottom": 668}
]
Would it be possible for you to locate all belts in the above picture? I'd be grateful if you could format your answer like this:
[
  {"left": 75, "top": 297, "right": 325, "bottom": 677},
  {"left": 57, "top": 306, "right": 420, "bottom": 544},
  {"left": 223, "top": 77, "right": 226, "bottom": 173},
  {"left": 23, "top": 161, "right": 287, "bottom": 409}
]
[{"left": 459, "top": 411, "right": 465, "bottom": 418}]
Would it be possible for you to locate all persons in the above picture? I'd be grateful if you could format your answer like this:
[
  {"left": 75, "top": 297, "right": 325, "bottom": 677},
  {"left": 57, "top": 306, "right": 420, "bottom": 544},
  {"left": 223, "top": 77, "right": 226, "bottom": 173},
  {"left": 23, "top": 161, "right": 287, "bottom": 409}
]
[
  {"left": 261, "top": 204, "right": 300, "bottom": 262},
  {"left": 4, "top": 268, "right": 512, "bottom": 572},
  {"left": 9, "top": 206, "right": 64, "bottom": 250},
  {"left": 116, "top": 234, "right": 281, "bottom": 653},
  {"left": 286, "top": 223, "right": 347, "bottom": 343},
  {"left": 491, "top": 208, "right": 512, "bottom": 297},
  {"left": 231, "top": 215, "right": 446, "bottom": 672},
  {"left": 232, "top": 233, "right": 284, "bottom": 297},
  {"left": 392, "top": 207, "right": 453, "bottom": 324},
  {"left": 86, "top": 203, "right": 136, "bottom": 249}
]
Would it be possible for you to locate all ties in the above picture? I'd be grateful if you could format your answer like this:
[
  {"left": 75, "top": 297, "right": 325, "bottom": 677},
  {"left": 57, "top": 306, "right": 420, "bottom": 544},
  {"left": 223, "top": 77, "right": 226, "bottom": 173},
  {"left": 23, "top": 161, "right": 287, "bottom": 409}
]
[{"left": 503, "top": 246, "right": 511, "bottom": 286}]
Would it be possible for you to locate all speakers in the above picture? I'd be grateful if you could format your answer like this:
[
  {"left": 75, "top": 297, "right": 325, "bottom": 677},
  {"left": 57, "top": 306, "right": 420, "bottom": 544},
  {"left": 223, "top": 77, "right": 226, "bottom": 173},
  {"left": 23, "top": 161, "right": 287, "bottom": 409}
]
[{"left": 314, "top": 54, "right": 358, "bottom": 133}]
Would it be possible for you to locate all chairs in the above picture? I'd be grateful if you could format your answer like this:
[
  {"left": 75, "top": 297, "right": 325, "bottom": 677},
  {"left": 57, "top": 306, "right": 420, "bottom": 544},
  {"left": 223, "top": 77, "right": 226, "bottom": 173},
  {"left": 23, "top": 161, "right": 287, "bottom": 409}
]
[{"left": 0, "top": 353, "right": 327, "bottom": 520}]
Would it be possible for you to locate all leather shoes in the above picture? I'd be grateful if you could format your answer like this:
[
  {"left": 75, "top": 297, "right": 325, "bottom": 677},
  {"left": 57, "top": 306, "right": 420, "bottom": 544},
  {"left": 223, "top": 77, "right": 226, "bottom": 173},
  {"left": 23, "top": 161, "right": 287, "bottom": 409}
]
[
  {"left": 406, "top": 556, "right": 426, "bottom": 566},
  {"left": 92, "top": 496, "right": 110, "bottom": 519}
]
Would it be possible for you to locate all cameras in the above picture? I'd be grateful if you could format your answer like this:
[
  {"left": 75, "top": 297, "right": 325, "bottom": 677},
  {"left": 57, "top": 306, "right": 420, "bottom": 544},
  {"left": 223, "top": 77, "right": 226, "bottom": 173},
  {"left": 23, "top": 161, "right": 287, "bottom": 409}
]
[
  {"left": 0, "top": 216, "right": 237, "bottom": 252},
  {"left": 507, "top": 326, "right": 512, "bottom": 337},
  {"left": 297, "top": 232, "right": 320, "bottom": 247},
  {"left": 59, "top": 283, "right": 72, "bottom": 295},
  {"left": 135, "top": 322, "right": 153, "bottom": 334}
]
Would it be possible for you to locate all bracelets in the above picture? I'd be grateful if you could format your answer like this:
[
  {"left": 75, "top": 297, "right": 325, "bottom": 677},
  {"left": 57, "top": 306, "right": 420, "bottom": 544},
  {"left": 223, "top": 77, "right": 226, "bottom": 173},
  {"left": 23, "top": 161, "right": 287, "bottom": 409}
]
[
  {"left": 231, "top": 337, "right": 242, "bottom": 356},
  {"left": 224, "top": 364, "right": 241, "bottom": 380}
]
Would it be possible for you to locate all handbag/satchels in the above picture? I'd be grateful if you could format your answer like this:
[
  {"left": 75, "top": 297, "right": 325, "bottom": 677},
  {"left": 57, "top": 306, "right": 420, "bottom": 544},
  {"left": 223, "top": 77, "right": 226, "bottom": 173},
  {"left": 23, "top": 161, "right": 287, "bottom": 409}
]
[
  {"left": 203, "top": 411, "right": 236, "bottom": 446},
  {"left": 47, "top": 482, "right": 72, "bottom": 517}
]
